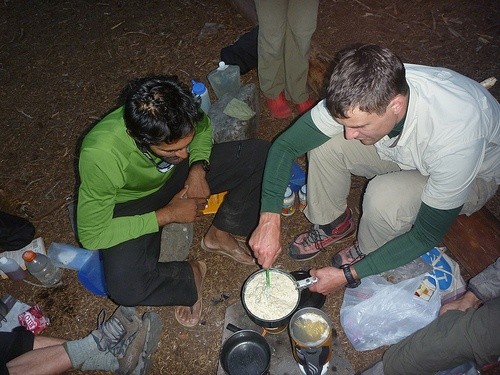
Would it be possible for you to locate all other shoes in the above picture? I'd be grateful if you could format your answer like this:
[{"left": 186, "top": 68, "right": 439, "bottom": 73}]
[
  {"left": 296, "top": 97, "right": 315, "bottom": 115},
  {"left": 267, "top": 93, "right": 292, "bottom": 118}
]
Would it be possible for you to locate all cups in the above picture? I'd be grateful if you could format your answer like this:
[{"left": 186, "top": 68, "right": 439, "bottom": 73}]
[{"left": 289, "top": 306, "right": 333, "bottom": 375}]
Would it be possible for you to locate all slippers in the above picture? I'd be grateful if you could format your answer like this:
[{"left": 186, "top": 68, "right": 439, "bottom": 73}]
[
  {"left": 175, "top": 260, "right": 208, "bottom": 327},
  {"left": 201, "top": 236, "right": 257, "bottom": 266}
]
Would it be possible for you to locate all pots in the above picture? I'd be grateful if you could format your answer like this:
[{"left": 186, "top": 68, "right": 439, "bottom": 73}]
[
  {"left": 220, "top": 329, "right": 272, "bottom": 375},
  {"left": 241, "top": 268, "right": 319, "bottom": 328}
]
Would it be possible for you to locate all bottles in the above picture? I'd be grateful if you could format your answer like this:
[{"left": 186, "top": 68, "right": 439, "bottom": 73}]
[
  {"left": 281, "top": 187, "right": 296, "bottom": 216},
  {"left": 0, "top": 257, "right": 26, "bottom": 281},
  {"left": 299, "top": 185, "right": 307, "bottom": 213},
  {"left": 22, "top": 251, "right": 61, "bottom": 285},
  {"left": 191, "top": 80, "right": 211, "bottom": 115}
]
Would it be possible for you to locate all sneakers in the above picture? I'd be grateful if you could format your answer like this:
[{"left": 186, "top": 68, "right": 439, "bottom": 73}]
[
  {"left": 332, "top": 241, "right": 366, "bottom": 268},
  {"left": 91, "top": 306, "right": 142, "bottom": 353},
  {"left": 287, "top": 208, "right": 358, "bottom": 262},
  {"left": 115, "top": 310, "right": 162, "bottom": 375}
]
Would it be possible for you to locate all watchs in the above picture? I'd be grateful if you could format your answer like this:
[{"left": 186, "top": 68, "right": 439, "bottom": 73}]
[
  {"left": 190, "top": 160, "right": 211, "bottom": 172},
  {"left": 340, "top": 264, "right": 361, "bottom": 289}
]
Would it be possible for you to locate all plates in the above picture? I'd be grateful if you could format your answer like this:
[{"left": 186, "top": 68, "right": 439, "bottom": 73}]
[{"left": 290, "top": 271, "right": 326, "bottom": 310}]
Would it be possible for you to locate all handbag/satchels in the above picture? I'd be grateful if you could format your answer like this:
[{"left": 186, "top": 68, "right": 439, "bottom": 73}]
[{"left": 339, "top": 247, "right": 467, "bottom": 351}]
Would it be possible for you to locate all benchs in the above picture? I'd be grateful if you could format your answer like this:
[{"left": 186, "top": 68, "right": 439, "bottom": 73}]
[{"left": 442, "top": 204, "right": 500, "bottom": 282}]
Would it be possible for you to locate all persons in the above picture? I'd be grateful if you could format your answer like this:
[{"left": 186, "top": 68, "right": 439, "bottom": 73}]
[
  {"left": 248, "top": 45, "right": 500, "bottom": 296},
  {"left": 360, "top": 256, "right": 500, "bottom": 375},
  {"left": 76, "top": 75, "right": 272, "bottom": 327},
  {"left": 0, "top": 304, "right": 160, "bottom": 375},
  {"left": 256, "top": 0, "right": 318, "bottom": 118}
]
